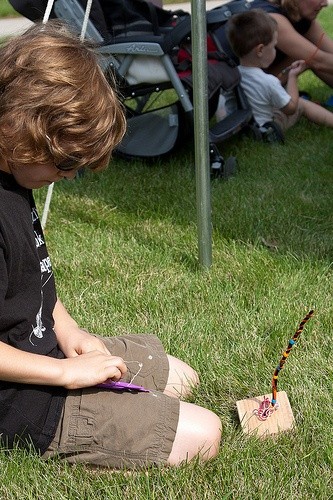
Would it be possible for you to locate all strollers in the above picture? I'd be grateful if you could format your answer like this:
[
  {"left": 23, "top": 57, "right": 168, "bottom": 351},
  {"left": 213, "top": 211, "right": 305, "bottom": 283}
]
[{"left": 10, "top": 0, "right": 287, "bottom": 183}]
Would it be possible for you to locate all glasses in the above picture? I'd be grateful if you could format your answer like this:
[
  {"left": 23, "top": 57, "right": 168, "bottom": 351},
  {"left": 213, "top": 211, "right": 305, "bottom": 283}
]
[{"left": 48, "top": 137, "right": 81, "bottom": 170}]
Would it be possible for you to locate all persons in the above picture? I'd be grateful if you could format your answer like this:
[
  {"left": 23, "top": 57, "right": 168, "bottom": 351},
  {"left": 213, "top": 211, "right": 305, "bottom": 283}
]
[
  {"left": 0, "top": 19, "right": 222, "bottom": 478},
  {"left": 207, "top": 1, "right": 333, "bottom": 145}
]
